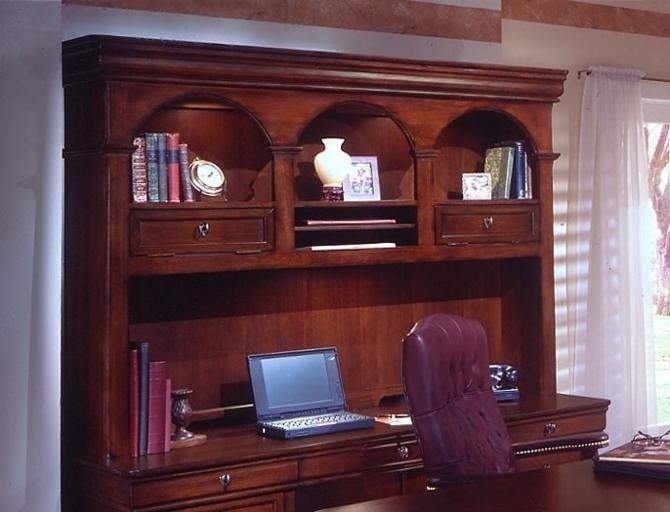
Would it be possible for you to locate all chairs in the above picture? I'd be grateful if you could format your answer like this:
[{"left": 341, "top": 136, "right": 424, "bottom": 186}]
[{"left": 400, "top": 312, "right": 613, "bottom": 483}]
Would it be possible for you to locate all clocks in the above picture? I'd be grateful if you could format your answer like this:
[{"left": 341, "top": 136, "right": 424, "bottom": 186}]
[{"left": 186, "top": 155, "right": 230, "bottom": 201}]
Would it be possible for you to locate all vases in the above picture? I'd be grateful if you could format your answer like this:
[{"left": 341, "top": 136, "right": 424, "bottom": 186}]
[{"left": 314, "top": 138, "right": 352, "bottom": 185}]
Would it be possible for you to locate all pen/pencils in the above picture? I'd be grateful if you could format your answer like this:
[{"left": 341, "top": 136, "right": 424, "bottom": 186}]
[{"left": 377, "top": 414, "right": 410, "bottom": 418}]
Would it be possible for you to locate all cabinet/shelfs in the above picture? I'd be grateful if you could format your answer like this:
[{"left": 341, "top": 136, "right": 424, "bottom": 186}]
[{"left": 62, "top": 33, "right": 570, "bottom": 457}]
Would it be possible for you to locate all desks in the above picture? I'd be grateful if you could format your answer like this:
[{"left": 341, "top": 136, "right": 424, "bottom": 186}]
[{"left": 62, "top": 388, "right": 612, "bottom": 510}]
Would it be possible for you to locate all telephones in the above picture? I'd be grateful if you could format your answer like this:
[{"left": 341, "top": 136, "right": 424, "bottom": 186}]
[{"left": 488, "top": 364, "right": 520, "bottom": 402}]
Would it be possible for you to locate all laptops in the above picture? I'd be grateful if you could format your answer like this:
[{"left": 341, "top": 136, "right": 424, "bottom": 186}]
[{"left": 245, "top": 345, "right": 375, "bottom": 439}]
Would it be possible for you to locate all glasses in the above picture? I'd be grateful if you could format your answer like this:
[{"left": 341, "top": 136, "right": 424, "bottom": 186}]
[{"left": 631, "top": 430, "right": 670, "bottom": 446}]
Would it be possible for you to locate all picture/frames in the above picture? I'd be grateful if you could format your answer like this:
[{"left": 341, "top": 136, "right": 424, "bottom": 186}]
[
  {"left": 462, "top": 172, "right": 492, "bottom": 200},
  {"left": 345, "top": 154, "right": 382, "bottom": 202}
]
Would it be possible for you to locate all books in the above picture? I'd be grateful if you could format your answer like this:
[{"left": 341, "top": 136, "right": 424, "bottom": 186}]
[
  {"left": 299, "top": 214, "right": 397, "bottom": 227},
  {"left": 296, "top": 240, "right": 398, "bottom": 253},
  {"left": 130, "top": 130, "right": 194, "bottom": 204},
  {"left": 596, "top": 435, "right": 670, "bottom": 480},
  {"left": 127, "top": 340, "right": 173, "bottom": 458},
  {"left": 483, "top": 138, "right": 534, "bottom": 203}
]
